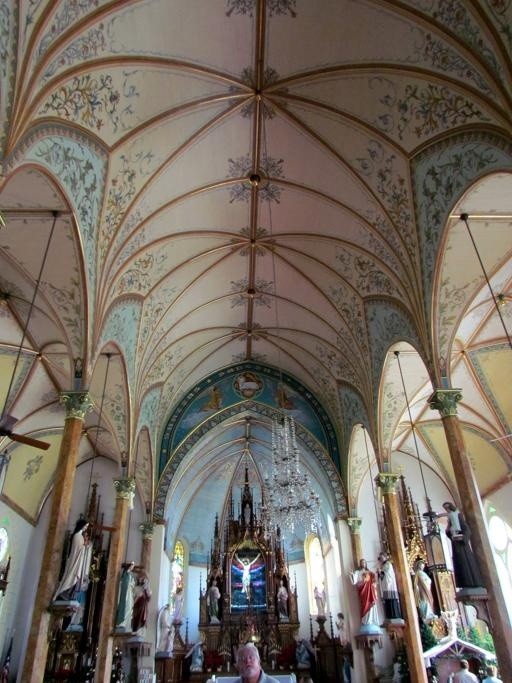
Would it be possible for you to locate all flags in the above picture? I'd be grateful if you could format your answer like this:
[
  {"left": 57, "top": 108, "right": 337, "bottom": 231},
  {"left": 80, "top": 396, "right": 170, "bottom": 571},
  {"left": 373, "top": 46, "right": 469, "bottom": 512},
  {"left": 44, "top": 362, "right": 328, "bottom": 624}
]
[{"left": 0, "top": 636, "right": 13, "bottom": 683}]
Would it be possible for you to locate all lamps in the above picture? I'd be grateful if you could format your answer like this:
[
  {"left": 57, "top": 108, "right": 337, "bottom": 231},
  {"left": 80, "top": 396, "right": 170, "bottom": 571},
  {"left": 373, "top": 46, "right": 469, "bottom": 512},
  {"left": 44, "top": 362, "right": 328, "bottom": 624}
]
[
  {"left": 396, "top": 352, "right": 448, "bottom": 574},
  {"left": 259, "top": 197, "right": 320, "bottom": 542}
]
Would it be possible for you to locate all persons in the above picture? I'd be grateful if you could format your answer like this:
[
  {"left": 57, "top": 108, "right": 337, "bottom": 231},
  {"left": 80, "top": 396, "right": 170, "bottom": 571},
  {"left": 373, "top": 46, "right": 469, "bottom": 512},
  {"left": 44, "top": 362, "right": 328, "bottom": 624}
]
[
  {"left": 442, "top": 501, "right": 484, "bottom": 588},
  {"left": 113, "top": 561, "right": 151, "bottom": 642},
  {"left": 314, "top": 581, "right": 327, "bottom": 615},
  {"left": 234, "top": 553, "right": 260, "bottom": 600},
  {"left": 429, "top": 658, "right": 504, "bottom": 683},
  {"left": 53, "top": 519, "right": 94, "bottom": 631},
  {"left": 233, "top": 644, "right": 281, "bottom": 683},
  {"left": 277, "top": 579, "right": 289, "bottom": 619},
  {"left": 189, "top": 644, "right": 208, "bottom": 672},
  {"left": 295, "top": 636, "right": 311, "bottom": 669},
  {"left": 392, "top": 657, "right": 404, "bottom": 683},
  {"left": 349, "top": 552, "right": 439, "bottom": 635},
  {"left": 335, "top": 612, "right": 349, "bottom": 647},
  {"left": 157, "top": 561, "right": 185, "bottom": 652},
  {"left": 206, "top": 580, "right": 221, "bottom": 624}
]
